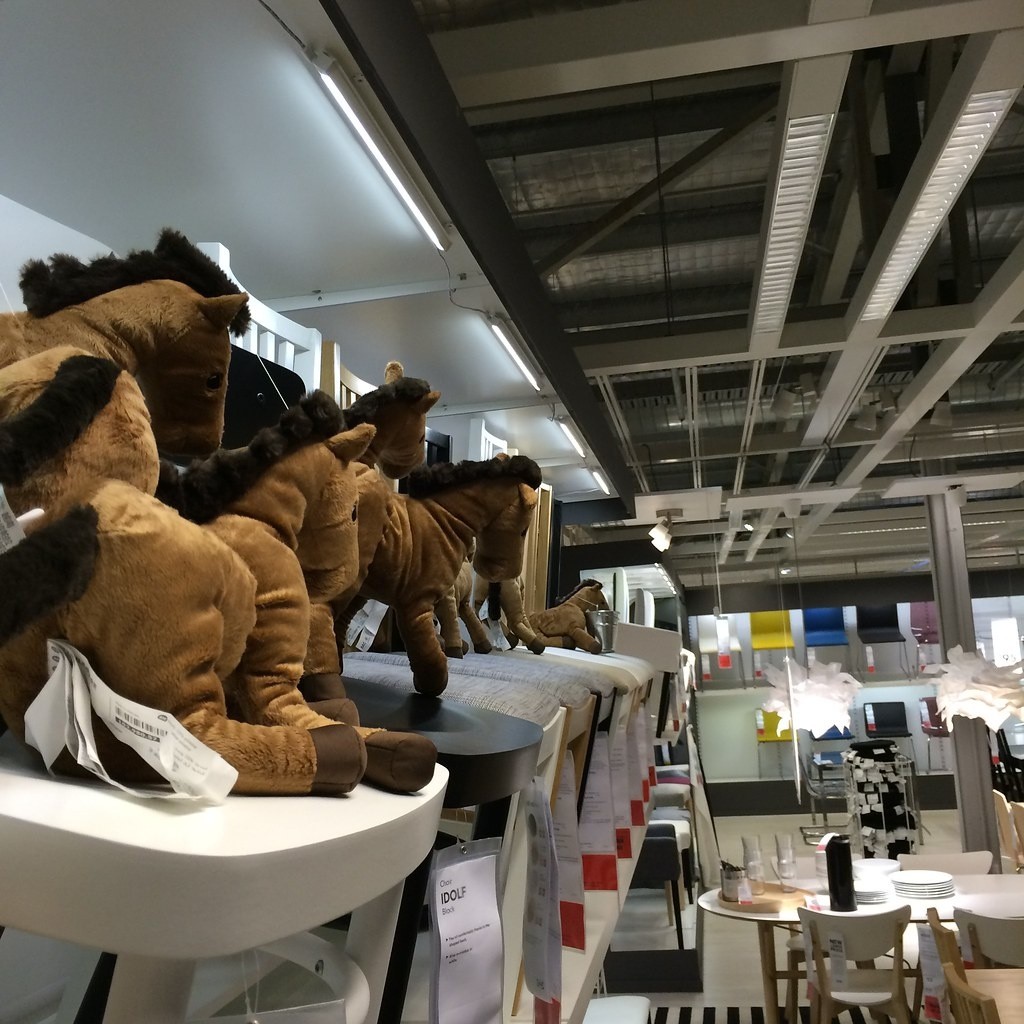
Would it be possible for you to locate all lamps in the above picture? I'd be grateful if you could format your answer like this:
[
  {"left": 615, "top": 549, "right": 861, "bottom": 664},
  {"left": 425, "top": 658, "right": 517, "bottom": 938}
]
[
  {"left": 760, "top": 499, "right": 863, "bottom": 742},
  {"left": 712, "top": 520, "right": 731, "bottom": 671},
  {"left": 310, "top": 51, "right": 453, "bottom": 254},
  {"left": 646, "top": 508, "right": 684, "bottom": 542},
  {"left": 651, "top": 532, "right": 673, "bottom": 554},
  {"left": 920, "top": 485, "right": 1023, "bottom": 733}
]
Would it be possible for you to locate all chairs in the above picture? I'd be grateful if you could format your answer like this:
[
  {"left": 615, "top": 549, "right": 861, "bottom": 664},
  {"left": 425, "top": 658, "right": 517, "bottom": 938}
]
[
  {"left": 910, "top": 602, "right": 943, "bottom": 678},
  {"left": 952, "top": 906, "right": 1023, "bottom": 970},
  {"left": 924, "top": 902, "right": 968, "bottom": 1023},
  {"left": 861, "top": 700, "right": 921, "bottom": 775},
  {"left": 802, "top": 606, "right": 857, "bottom": 684},
  {"left": 812, "top": 724, "right": 859, "bottom": 780},
  {"left": 756, "top": 708, "right": 803, "bottom": 777},
  {"left": 796, "top": 902, "right": 912, "bottom": 1023},
  {"left": 851, "top": 603, "right": 914, "bottom": 683},
  {"left": 0, "top": 239, "right": 653, "bottom": 1023},
  {"left": 897, "top": 850, "right": 993, "bottom": 875},
  {"left": 745, "top": 611, "right": 799, "bottom": 689},
  {"left": 991, "top": 787, "right": 1024, "bottom": 873},
  {"left": 942, "top": 959, "right": 1003, "bottom": 1024},
  {"left": 917, "top": 697, "right": 955, "bottom": 773},
  {"left": 695, "top": 613, "right": 748, "bottom": 692}
]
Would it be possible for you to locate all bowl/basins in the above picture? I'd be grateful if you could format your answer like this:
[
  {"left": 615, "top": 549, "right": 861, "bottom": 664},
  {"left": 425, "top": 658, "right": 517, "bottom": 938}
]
[{"left": 813, "top": 850, "right": 900, "bottom": 893}]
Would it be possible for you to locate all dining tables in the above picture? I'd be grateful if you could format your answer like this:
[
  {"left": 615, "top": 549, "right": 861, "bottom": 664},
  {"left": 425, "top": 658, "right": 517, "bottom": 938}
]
[{"left": 694, "top": 856, "right": 1023, "bottom": 1023}]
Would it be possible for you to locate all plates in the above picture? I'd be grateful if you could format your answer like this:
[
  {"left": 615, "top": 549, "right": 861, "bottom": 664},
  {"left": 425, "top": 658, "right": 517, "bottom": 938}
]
[{"left": 851, "top": 870, "right": 956, "bottom": 904}]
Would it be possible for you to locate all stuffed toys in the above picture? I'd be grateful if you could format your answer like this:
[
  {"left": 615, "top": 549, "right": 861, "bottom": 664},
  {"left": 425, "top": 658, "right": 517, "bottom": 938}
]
[
  {"left": 442, "top": 562, "right": 545, "bottom": 660},
  {"left": 0, "top": 392, "right": 436, "bottom": 798},
  {"left": 0, "top": 230, "right": 249, "bottom": 517},
  {"left": 336, "top": 454, "right": 543, "bottom": 697},
  {"left": 531, "top": 578, "right": 611, "bottom": 653},
  {"left": 305, "top": 360, "right": 441, "bottom": 725}
]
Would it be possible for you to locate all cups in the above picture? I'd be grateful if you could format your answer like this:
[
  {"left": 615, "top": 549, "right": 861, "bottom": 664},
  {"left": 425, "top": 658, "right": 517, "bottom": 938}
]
[{"left": 721, "top": 833, "right": 796, "bottom": 904}]
